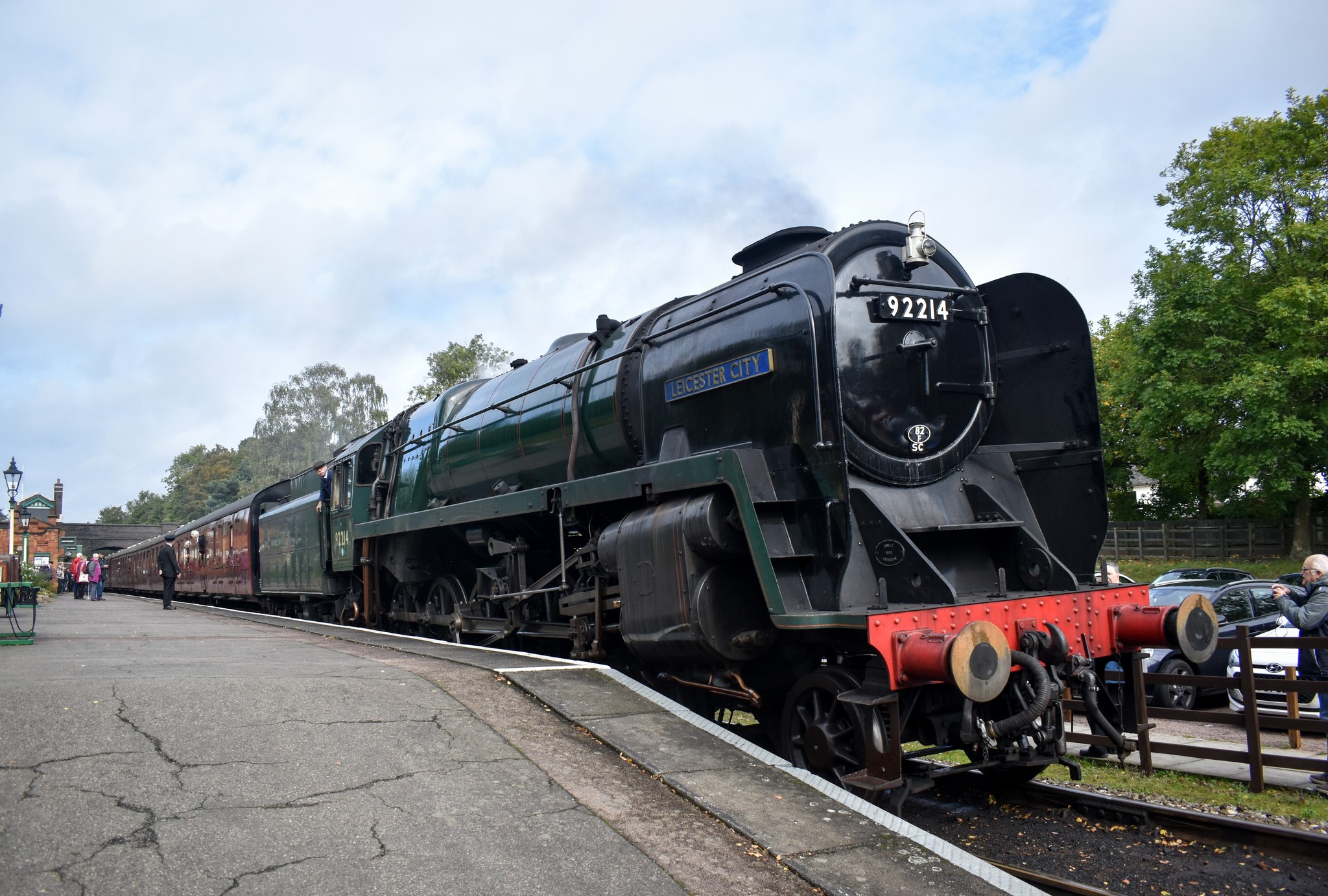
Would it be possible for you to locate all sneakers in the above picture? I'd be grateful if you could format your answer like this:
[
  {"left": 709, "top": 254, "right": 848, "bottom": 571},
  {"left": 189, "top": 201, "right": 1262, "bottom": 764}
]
[
  {"left": 1316, "top": 782, "right": 1328, "bottom": 793},
  {"left": 1309, "top": 773, "right": 1328, "bottom": 784},
  {"left": 98, "top": 598, "right": 106, "bottom": 600},
  {"left": 91, "top": 598, "right": 99, "bottom": 601}
]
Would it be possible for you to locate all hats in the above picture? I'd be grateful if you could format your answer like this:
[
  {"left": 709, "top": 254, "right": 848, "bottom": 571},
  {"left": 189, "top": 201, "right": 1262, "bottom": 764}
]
[
  {"left": 99, "top": 551, "right": 105, "bottom": 554},
  {"left": 313, "top": 460, "right": 326, "bottom": 469},
  {"left": 48, "top": 561, "right": 54, "bottom": 564},
  {"left": 164, "top": 534, "right": 176, "bottom": 542}
]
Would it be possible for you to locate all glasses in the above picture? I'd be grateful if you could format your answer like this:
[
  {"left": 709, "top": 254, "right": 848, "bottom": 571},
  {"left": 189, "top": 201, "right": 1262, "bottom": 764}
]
[
  {"left": 315, "top": 467, "right": 323, "bottom": 472},
  {"left": 1302, "top": 567, "right": 1319, "bottom": 573}
]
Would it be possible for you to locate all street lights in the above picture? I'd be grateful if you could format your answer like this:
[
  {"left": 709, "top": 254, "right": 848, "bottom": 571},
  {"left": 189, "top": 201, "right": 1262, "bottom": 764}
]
[
  {"left": 19, "top": 504, "right": 31, "bottom": 565},
  {"left": 2, "top": 455, "right": 23, "bottom": 554}
]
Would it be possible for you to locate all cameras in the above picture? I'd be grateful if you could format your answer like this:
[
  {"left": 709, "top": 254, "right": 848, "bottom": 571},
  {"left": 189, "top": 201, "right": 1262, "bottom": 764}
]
[{"left": 1270, "top": 586, "right": 1279, "bottom": 599}]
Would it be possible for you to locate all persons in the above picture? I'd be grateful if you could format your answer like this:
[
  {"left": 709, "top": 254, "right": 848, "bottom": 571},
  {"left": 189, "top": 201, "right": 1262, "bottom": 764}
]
[
  {"left": 313, "top": 460, "right": 332, "bottom": 514},
  {"left": 117, "top": 561, "right": 121, "bottom": 571},
  {"left": 57, "top": 561, "right": 74, "bottom": 594},
  {"left": 41, "top": 561, "right": 54, "bottom": 589},
  {"left": 71, "top": 551, "right": 110, "bottom": 601},
  {"left": 1079, "top": 561, "right": 1142, "bottom": 759},
  {"left": 184, "top": 540, "right": 192, "bottom": 559},
  {"left": 191, "top": 530, "right": 206, "bottom": 561},
  {"left": 128, "top": 558, "right": 132, "bottom": 571},
  {"left": 1271, "top": 553, "right": 1328, "bottom": 795},
  {"left": 157, "top": 534, "right": 181, "bottom": 610}
]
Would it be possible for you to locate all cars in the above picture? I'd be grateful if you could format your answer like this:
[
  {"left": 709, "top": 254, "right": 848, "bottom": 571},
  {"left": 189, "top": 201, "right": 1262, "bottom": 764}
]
[
  {"left": 1275, "top": 573, "right": 1308, "bottom": 588},
  {"left": 1072, "top": 577, "right": 1310, "bottom": 711},
  {"left": 1151, "top": 568, "right": 1256, "bottom": 584},
  {"left": 1094, "top": 569, "right": 1137, "bottom": 584},
  {"left": 1225, "top": 611, "right": 1322, "bottom": 718}
]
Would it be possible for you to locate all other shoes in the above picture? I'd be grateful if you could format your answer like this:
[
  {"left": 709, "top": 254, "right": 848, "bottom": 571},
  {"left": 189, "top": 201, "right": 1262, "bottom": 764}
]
[
  {"left": 63, "top": 592, "right": 68, "bottom": 594},
  {"left": 74, "top": 597, "right": 86, "bottom": 600},
  {"left": 57, "top": 592, "right": 63, "bottom": 594}
]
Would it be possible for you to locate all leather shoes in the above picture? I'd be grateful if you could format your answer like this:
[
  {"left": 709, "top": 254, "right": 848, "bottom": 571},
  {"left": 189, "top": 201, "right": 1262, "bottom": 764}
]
[{"left": 163, "top": 606, "right": 177, "bottom": 610}]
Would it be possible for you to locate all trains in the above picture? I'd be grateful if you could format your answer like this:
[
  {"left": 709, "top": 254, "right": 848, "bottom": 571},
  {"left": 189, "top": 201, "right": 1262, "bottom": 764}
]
[{"left": 101, "top": 219, "right": 1220, "bottom": 820}]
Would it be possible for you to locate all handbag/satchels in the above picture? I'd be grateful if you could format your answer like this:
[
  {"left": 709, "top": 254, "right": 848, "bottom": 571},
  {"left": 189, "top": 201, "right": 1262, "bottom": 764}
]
[
  {"left": 85, "top": 561, "right": 96, "bottom": 573},
  {"left": 57, "top": 566, "right": 64, "bottom": 579},
  {"left": 79, "top": 571, "right": 89, "bottom": 583}
]
